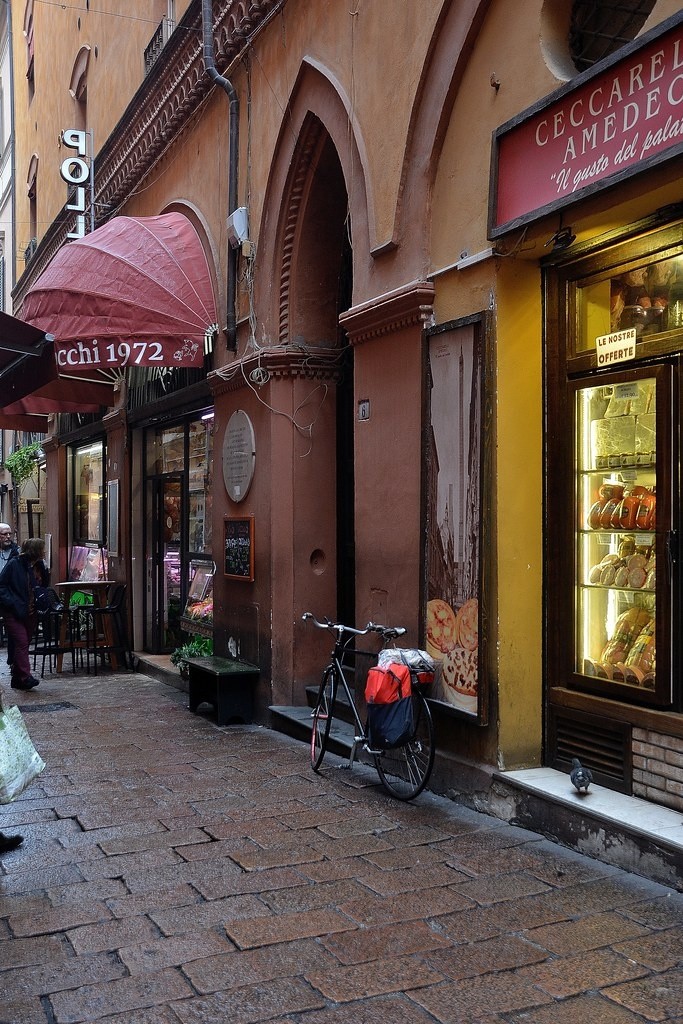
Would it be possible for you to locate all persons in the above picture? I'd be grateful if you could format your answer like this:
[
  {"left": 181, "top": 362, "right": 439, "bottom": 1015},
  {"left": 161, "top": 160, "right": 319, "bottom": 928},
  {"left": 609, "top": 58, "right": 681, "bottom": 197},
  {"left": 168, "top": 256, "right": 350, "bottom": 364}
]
[
  {"left": 0, "top": 690, "right": 24, "bottom": 853},
  {"left": 0, "top": 539, "right": 52, "bottom": 690},
  {"left": 0, "top": 522, "right": 22, "bottom": 575}
]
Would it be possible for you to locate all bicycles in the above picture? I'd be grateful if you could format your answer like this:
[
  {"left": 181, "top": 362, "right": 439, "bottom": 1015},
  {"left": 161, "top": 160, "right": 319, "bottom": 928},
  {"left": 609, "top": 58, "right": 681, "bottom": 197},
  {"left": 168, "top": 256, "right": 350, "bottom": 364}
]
[{"left": 300, "top": 610, "right": 438, "bottom": 803}]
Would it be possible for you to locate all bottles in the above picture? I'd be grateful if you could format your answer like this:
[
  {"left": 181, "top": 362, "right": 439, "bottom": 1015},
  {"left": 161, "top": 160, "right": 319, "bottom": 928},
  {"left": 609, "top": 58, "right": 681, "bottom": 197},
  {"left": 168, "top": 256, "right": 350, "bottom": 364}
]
[
  {"left": 627, "top": 606, "right": 658, "bottom": 674},
  {"left": 667, "top": 282, "right": 683, "bottom": 329},
  {"left": 601, "top": 602, "right": 651, "bottom": 665}
]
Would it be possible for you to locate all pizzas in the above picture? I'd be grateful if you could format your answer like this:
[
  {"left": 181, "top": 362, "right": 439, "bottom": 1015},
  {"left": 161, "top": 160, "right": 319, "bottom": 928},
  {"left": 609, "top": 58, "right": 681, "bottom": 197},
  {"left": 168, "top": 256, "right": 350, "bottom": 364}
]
[{"left": 424, "top": 597, "right": 477, "bottom": 696}]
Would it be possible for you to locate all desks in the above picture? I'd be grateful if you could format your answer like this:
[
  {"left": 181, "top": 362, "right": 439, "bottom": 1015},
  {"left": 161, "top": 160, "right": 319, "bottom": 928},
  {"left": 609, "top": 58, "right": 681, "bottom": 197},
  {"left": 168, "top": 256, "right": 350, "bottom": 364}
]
[{"left": 54, "top": 581, "right": 118, "bottom": 673}]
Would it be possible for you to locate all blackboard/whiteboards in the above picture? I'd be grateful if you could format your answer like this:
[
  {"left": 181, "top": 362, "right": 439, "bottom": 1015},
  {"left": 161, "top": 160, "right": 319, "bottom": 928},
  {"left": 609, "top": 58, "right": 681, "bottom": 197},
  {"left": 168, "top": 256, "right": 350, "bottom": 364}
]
[{"left": 222, "top": 516, "right": 255, "bottom": 582}]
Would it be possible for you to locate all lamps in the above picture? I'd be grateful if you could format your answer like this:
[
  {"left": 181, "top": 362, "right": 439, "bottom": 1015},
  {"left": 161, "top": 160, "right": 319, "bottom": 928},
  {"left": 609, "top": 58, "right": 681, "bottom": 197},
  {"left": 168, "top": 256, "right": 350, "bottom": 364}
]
[{"left": 544, "top": 212, "right": 577, "bottom": 254}]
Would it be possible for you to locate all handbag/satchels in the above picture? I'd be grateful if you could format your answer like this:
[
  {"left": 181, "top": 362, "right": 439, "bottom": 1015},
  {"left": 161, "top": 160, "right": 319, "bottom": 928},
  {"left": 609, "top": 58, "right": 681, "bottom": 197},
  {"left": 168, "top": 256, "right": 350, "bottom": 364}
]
[
  {"left": 33, "top": 587, "right": 52, "bottom": 616},
  {"left": 365, "top": 647, "right": 435, "bottom": 749}
]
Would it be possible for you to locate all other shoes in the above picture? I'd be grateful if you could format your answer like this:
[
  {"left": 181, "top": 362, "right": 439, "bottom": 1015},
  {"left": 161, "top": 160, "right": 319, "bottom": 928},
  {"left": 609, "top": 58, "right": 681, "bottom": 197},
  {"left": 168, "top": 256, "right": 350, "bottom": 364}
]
[
  {"left": 10, "top": 680, "right": 27, "bottom": 690},
  {"left": 22, "top": 676, "right": 39, "bottom": 689}
]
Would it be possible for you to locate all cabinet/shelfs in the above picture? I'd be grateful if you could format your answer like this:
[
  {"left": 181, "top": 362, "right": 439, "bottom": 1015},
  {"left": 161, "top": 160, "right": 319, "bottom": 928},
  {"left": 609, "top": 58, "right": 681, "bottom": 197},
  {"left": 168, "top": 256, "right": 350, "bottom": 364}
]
[{"left": 574, "top": 373, "right": 656, "bottom": 689}]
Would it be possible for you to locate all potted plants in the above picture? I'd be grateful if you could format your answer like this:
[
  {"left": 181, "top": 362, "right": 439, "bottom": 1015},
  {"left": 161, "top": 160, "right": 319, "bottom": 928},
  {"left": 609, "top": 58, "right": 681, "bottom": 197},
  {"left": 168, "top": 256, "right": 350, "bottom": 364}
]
[{"left": 170, "top": 634, "right": 210, "bottom": 681}]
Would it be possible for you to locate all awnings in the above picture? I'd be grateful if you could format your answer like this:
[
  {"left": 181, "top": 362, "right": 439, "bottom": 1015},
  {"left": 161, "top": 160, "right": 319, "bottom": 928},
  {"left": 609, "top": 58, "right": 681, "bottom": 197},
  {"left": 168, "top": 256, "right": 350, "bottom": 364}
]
[
  {"left": 1, "top": 211, "right": 217, "bottom": 434},
  {"left": 0, "top": 312, "right": 57, "bottom": 408}
]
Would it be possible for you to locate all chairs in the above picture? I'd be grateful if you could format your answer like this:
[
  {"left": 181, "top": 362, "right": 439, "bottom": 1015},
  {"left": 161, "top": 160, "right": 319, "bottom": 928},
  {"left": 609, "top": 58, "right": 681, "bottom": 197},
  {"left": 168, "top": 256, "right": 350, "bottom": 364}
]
[{"left": 29, "top": 583, "right": 135, "bottom": 678}]
